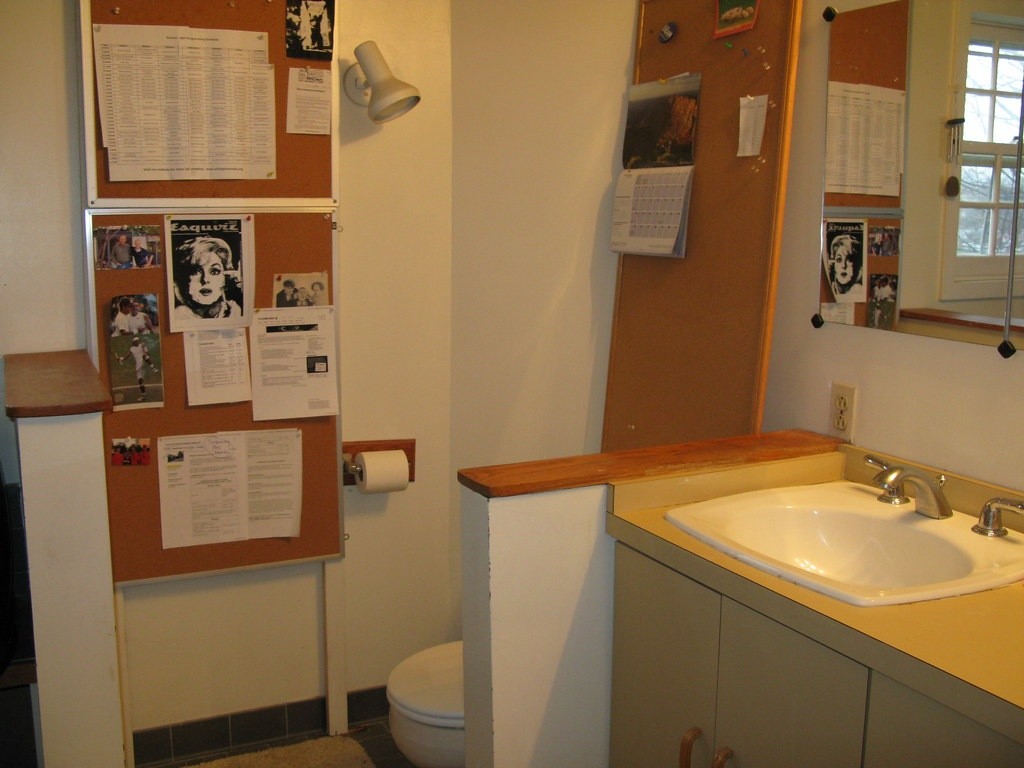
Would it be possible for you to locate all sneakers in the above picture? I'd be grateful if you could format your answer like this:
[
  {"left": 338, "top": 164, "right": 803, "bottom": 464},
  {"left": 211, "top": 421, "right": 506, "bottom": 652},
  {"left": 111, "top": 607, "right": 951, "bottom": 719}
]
[
  {"left": 138, "top": 393, "right": 146, "bottom": 401},
  {"left": 152, "top": 369, "right": 158, "bottom": 373}
]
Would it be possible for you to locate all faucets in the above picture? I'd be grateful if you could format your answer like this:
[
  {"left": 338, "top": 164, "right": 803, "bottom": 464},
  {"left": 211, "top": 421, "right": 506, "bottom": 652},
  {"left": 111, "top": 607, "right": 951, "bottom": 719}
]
[{"left": 873, "top": 466, "right": 953, "bottom": 519}]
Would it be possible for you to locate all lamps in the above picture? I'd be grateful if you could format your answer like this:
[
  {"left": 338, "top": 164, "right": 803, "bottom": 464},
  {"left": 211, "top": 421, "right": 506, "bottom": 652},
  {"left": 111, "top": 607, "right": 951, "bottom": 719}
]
[{"left": 343, "top": 40, "right": 422, "bottom": 124}]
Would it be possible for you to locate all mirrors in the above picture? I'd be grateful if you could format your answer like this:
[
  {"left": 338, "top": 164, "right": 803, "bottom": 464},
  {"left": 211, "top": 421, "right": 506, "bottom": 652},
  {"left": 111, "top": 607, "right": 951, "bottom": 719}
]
[{"left": 810, "top": 0, "right": 1024, "bottom": 358}]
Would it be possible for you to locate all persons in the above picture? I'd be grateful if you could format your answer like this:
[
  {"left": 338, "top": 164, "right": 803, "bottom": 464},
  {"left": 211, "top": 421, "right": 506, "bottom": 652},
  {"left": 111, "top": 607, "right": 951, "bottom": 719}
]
[
  {"left": 866, "top": 226, "right": 901, "bottom": 332},
  {"left": 110, "top": 233, "right": 163, "bottom": 466},
  {"left": 277, "top": 280, "right": 325, "bottom": 307}
]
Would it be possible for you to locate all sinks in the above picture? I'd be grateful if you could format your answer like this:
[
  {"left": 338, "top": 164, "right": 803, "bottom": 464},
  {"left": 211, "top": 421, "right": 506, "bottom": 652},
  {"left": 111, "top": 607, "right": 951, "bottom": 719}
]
[{"left": 663, "top": 480, "right": 1023, "bottom": 606}]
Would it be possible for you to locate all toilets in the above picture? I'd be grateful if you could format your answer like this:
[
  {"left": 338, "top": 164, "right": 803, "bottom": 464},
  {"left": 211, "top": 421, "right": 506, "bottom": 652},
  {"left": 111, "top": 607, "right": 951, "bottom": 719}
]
[{"left": 386, "top": 641, "right": 464, "bottom": 768}]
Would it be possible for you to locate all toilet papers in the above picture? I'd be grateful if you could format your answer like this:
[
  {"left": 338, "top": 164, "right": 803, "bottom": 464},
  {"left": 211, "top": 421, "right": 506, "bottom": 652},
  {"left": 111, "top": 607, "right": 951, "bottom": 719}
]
[{"left": 355, "top": 449, "right": 408, "bottom": 494}]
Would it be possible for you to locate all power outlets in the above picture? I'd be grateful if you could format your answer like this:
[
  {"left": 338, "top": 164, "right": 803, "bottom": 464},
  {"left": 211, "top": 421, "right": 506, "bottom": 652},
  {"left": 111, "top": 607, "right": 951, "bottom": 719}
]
[{"left": 829, "top": 380, "right": 857, "bottom": 444}]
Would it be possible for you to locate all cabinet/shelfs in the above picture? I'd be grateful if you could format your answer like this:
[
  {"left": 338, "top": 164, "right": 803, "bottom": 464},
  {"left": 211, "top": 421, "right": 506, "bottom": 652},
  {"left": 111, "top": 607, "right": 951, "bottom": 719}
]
[
  {"left": 862, "top": 664, "right": 1024, "bottom": 768},
  {"left": 594, "top": 512, "right": 870, "bottom": 768}
]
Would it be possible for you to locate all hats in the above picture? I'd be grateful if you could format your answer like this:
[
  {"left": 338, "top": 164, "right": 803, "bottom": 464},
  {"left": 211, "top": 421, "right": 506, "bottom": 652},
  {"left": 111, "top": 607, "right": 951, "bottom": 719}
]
[
  {"left": 121, "top": 298, "right": 134, "bottom": 304},
  {"left": 133, "top": 337, "right": 139, "bottom": 341}
]
